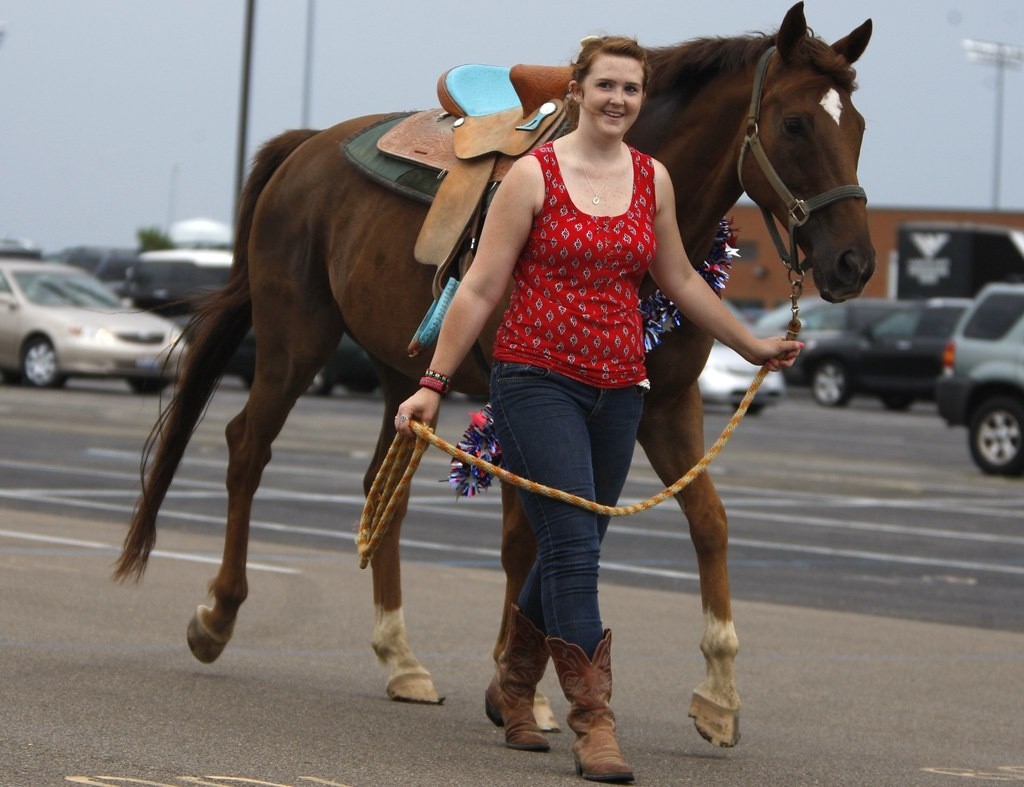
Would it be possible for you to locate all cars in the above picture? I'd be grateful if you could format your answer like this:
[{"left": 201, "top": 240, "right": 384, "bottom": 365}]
[
  {"left": 800, "top": 298, "right": 972, "bottom": 413},
  {"left": 700, "top": 341, "right": 786, "bottom": 417},
  {"left": 1, "top": 259, "right": 189, "bottom": 396},
  {"left": 123, "top": 250, "right": 375, "bottom": 400},
  {"left": 936, "top": 281, "right": 1023, "bottom": 480},
  {"left": 753, "top": 295, "right": 888, "bottom": 387}
]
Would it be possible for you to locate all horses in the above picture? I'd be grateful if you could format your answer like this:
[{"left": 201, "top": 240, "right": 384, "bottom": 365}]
[{"left": 103, "top": 0, "right": 881, "bottom": 751}]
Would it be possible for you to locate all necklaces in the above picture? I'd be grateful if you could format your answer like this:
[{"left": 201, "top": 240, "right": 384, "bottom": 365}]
[{"left": 572, "top": 140, "right": 622, "bottom": 204}]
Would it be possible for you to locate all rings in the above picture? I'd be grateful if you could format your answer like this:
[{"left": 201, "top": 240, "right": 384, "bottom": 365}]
[
  {"left": 400, "top": 416, "right": 408, "bottom": 423},
  {"left": 394, "top": 416, "right": 398, "bottom": 420}
]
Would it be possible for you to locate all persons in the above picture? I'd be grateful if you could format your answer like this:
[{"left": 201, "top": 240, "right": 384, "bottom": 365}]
[{"left": 395, "top": 37, "right": 805, "bottom": 781}]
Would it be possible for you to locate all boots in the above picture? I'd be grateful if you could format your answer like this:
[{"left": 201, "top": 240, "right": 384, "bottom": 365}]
[
  {"left": 486, "top": 603, "right": 554, "bottom": 750},
  {"left": 547, "top": 628, "right": 636, "bottom": 782}
]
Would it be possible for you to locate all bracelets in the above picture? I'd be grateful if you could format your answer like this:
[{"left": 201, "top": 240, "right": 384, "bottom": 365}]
[{"left": 419, "top": 369, "right": 452, "bottom": 396}]
